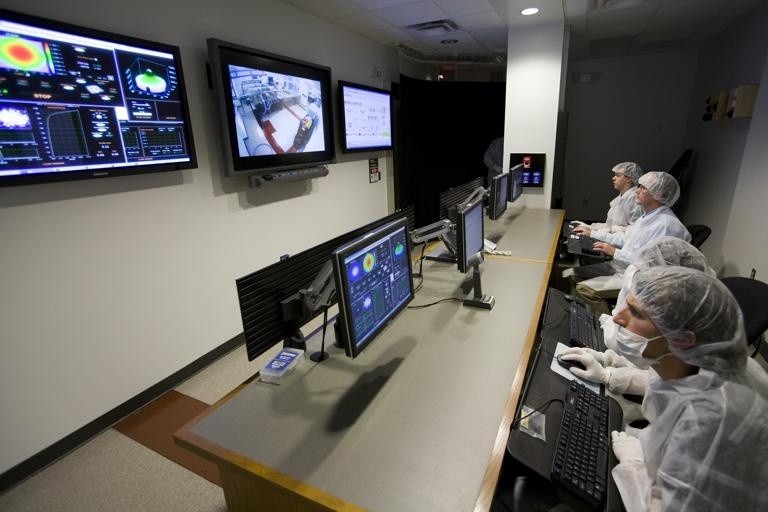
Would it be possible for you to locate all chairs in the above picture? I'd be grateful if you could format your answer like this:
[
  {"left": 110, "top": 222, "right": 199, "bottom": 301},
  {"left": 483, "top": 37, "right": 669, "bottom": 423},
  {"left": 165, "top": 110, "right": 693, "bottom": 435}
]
[
  {"left": 687, "top": 223, "right": 712, "bottom": 248},
  {"left": 720, "top": 275, "right": 767, "bottom": 345}
]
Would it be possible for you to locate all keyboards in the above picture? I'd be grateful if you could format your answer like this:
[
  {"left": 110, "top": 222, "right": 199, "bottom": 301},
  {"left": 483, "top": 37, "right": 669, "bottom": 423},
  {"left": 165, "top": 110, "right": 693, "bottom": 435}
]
[
  {"left": 578, "top": 234, "right": 604, "bottom": 256},
  {"left": 549, "top": 379, "right": 609, "bottom": 504},
  {"left": 569, "top": 300, "right": 599, "bottom": 351}
]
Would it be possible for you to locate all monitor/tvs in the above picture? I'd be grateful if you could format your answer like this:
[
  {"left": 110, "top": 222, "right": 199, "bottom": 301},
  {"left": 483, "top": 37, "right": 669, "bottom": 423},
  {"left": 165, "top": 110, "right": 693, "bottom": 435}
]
[
  {"left": 331, "top": 217, "right": 415, "bottom": 359},
  {"left": 457, "top": 197, "right": 484, "bottom": 273},
  {"left": 511, "top": 164, "right": 524, "bottom": 203},
  {"left": 337, "top": 79, "right": 391, "bottom": 154},
  {"left": 0, "top": 7, "right": 199, "bottom": 187},
  {"left": 207, "top": 37, "right": 337, "bottom": 177},
  {"left": 490, "top": 172, "right": 508, "bottom": 219}
]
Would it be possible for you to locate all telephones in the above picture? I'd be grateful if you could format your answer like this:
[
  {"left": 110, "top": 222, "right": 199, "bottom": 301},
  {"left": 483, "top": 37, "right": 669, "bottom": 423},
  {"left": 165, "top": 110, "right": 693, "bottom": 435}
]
[{"left": 484, "top": 239, "right": 496, "bottom": 252}]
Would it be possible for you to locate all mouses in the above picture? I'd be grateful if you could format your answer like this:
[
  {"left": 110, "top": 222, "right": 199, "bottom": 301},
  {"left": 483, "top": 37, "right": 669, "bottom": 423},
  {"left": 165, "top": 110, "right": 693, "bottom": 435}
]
[
  {"left": 564, "top": 294, "right": 584, "bottom": 306},
  {"left": 557, "top": 354, "right": 585, "bottom": 371}
]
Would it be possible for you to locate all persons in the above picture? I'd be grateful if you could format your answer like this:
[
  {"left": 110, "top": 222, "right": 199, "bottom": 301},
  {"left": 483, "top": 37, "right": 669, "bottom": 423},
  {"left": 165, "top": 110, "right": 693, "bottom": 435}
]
[
  {"left": 484, "top": 136, "right": 503, "bottom": 191},
  {"left": 558, "top": 265, "right": 768, "bottom": 511},
  {"left": 579, "top": 233, "right": 768, "bottom": 398},
  {"left": 568, "top": 161, "right": 647, "bottom": 268},
  {"left": 561, "top": 170, "right": 692, "bottom": 317}
]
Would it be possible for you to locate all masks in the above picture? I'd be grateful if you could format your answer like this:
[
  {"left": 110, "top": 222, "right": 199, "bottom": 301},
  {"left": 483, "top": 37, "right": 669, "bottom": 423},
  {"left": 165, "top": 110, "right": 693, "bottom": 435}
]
[{"left": 617, "top": 326, "right": 685, "bottom": 370}]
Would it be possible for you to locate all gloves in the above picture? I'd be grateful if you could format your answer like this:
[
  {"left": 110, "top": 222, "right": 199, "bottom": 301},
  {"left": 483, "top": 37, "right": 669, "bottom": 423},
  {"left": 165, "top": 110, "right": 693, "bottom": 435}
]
[
  {"left": 570, "top": 221, "right": 591, "bottom": 229},
  {"left": 611, "top": 430, "right": 643, "bottom": 462},
  {"left": 561, "top": 347, "right": 610, "bottom": 384}
]
